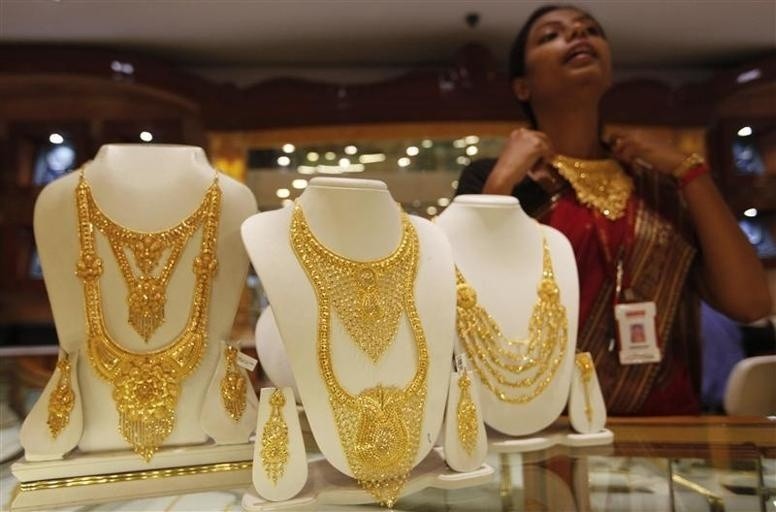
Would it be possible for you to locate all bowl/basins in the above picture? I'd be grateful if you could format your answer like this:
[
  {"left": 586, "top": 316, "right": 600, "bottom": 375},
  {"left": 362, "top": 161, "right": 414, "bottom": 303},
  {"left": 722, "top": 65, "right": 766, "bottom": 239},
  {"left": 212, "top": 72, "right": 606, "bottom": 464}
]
[
  {"left": 291, "top": 197, "right": 430, "bottom": 508},
  {"left": 542, "top": 148, "right": 637, "bottom": 218},
  {"left": 76, "top": 167, "right": 222, "bottom": 464},
  {"left": 456, "top": 227, "right": 567, "bottom": 404}
]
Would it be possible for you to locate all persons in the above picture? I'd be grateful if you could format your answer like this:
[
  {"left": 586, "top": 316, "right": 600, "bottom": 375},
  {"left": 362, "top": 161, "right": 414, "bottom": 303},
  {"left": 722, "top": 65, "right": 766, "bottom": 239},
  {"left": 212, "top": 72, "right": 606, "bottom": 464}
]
[{"left": 457, "top": 4, "right": 776, "bottom": 416}]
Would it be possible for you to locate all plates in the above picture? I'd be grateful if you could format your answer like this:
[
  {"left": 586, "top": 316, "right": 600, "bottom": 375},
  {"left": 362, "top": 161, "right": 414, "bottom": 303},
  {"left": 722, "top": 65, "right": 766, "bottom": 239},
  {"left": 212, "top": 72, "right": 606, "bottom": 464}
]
[{"left": 21, "top": 411, "right": 775, "bottom": 511}]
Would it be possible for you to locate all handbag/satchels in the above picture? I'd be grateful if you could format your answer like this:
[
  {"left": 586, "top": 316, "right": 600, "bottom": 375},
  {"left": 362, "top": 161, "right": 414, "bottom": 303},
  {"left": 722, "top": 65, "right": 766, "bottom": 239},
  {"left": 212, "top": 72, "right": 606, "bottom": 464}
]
[{"left": 673, "top": 152, "right": 708, "bottom": 185}]
